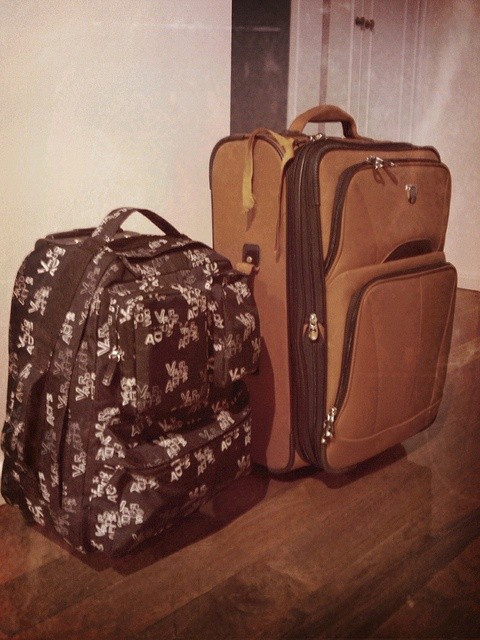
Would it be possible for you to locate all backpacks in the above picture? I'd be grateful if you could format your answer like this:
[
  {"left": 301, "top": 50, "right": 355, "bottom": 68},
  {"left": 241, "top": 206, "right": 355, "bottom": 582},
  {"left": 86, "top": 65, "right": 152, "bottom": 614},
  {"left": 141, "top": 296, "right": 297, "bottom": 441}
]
[{"left": 1, "top": 206, "right": 261, "bottom": 557}]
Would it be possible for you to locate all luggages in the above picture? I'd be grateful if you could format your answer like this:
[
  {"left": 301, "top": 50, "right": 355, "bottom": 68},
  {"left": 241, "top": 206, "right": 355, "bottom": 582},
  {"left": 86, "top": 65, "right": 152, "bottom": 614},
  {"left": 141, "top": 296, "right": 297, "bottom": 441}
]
[{"left": 209, "top": 105, "right": 457, "bottom": 475}]
